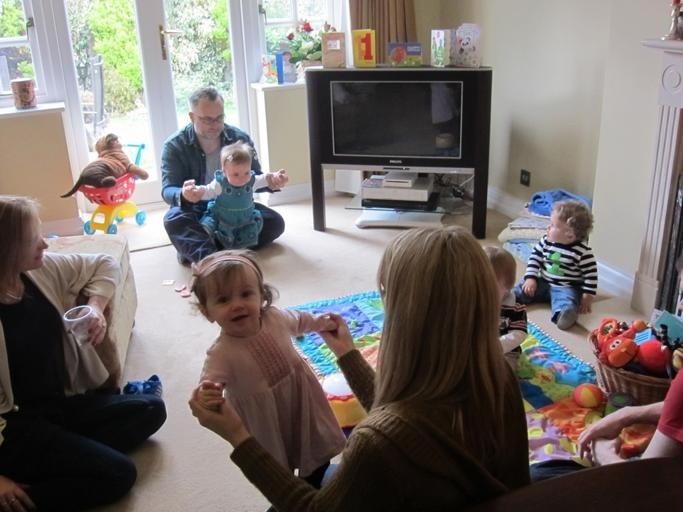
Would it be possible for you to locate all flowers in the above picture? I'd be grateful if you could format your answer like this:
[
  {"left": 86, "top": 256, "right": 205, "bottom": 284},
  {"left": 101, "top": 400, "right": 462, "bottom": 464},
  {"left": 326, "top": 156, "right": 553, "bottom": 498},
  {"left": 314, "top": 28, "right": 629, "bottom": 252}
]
[{"left": 280, "top": 18, "right": 339, "bottom": 67}]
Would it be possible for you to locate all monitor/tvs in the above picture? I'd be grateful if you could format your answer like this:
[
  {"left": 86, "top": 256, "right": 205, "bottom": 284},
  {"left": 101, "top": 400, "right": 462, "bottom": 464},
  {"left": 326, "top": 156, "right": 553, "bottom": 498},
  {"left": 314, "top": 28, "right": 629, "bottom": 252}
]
[{"left": 323, "top": 79, "right": 471, "bottom": 167}]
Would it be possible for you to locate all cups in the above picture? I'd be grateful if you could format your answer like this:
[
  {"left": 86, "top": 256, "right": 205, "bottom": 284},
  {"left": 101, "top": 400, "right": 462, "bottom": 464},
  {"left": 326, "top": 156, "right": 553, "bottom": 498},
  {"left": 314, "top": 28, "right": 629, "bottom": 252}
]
[
  {"left": 62, "top": 305, "right": 97, "bottom": 344},
  {"left": 9, "top": 77, "right": 37, "bottom": 110}
]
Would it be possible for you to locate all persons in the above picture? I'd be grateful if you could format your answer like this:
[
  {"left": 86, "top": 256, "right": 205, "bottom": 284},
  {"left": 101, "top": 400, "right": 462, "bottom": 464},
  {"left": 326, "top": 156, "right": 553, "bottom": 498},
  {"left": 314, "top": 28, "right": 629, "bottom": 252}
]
[
  {"left": 158, "top": 89, "right": 290, "bottom": 261},
  {"left": 508, "top": 199, "right": 605, "bottom": 332},
  {"left": 185, "top": 254, "right": 351, "bottom": 512},
  {"left": 527, "top": 341, "right": 683, "bottom": 488},
  {"left": 0, "top": 198, "right": 168, "bottom": 512},
  {"left": 189, "top": 226, "right": 531, "bottom": 511},
  {"left": 482, "top": 245, "right": 528, "bottom": 378},
  {"left": 187, "top": 140, "right": 277, "bottom": 250}
]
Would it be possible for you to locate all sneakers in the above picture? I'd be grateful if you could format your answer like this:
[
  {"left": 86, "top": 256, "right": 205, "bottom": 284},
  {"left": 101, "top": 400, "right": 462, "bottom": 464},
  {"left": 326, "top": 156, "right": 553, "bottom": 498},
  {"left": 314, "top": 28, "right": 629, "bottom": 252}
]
[{"left": 123, "top": 375, "right": 162, "bottom": 397}]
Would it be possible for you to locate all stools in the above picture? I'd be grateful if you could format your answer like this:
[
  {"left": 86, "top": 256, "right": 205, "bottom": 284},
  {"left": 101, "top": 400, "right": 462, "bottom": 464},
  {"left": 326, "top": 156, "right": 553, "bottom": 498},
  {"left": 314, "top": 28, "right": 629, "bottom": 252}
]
[{"left": 36, "top": 229, "right": 139, "bottom": 388}]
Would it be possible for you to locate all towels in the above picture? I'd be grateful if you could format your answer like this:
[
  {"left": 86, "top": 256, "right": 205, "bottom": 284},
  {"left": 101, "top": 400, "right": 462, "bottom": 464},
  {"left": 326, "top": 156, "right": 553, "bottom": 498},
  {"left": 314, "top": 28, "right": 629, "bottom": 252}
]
[
  {"left": 496, "top": 214, "right": 575, "bottom": 262},
  {"left": 523, "top": 189, "right": 591, "bottom": 215}
]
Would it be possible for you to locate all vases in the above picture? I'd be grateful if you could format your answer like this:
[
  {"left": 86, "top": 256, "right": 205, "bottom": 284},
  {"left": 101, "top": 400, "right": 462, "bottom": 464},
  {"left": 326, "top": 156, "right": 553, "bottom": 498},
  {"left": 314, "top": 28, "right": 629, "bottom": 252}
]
[{"left": 293, "top": 56, "right": 323, "bottom": 85}]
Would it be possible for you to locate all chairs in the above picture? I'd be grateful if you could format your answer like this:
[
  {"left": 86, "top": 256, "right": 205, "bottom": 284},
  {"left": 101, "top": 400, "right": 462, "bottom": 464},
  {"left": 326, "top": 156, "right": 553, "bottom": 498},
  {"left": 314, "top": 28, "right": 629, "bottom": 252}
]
[{"left": 81, "top": 52, "right": 110, "bottom": 151}]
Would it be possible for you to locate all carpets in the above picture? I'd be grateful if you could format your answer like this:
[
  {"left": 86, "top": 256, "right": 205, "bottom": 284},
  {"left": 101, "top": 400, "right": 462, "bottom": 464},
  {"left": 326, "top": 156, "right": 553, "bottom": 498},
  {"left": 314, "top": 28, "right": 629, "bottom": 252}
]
[{"left": 275, "top": 287, "right": 607, "bottom": 473}]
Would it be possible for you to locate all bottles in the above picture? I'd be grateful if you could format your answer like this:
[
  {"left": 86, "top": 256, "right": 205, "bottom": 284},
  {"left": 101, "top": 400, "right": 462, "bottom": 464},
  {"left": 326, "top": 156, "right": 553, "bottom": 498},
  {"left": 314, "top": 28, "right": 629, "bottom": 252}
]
[{"left": 661, "top": 1, "right": 682, "bottom": 41}]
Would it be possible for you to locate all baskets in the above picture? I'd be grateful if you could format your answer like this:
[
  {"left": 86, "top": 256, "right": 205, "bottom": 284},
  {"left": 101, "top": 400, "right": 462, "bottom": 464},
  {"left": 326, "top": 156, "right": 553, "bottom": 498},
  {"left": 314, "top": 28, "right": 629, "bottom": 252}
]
[{"left": 588, "top": 324, "right": 674, "bottom": 405}]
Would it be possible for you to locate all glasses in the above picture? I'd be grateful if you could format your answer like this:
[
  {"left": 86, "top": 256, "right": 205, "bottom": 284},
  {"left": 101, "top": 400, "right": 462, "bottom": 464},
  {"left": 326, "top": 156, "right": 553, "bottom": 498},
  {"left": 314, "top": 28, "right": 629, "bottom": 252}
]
[{"left": 198, "top": 113, "right": 225, "bottom": 124}]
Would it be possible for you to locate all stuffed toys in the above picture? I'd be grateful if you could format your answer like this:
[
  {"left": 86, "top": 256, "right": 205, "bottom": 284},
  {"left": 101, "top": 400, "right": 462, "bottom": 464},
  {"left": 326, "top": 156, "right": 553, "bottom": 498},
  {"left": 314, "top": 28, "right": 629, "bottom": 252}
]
[{"left": 592, "top": 310, "right": 683, "bottom": 374}]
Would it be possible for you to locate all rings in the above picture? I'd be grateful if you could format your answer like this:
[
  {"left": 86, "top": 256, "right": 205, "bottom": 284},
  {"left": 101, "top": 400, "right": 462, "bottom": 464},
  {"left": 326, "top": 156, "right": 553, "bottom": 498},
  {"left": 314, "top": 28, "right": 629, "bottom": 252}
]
[
  {"left": 9, "top": 497, "right": 16, "bottom": 505},
  {"left": 1, "top": 502, "right": 7, "bottom": 507}
]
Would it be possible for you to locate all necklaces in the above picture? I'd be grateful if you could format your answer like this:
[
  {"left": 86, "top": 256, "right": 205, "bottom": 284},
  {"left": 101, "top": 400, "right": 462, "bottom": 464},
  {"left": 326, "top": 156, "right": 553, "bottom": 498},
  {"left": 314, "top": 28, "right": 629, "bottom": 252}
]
[{"left": 4, "top": 285, "right": 27, "bottom": 307}]
